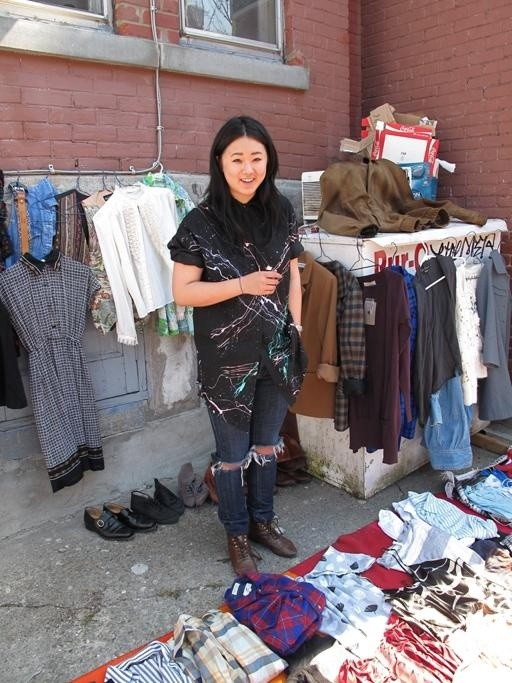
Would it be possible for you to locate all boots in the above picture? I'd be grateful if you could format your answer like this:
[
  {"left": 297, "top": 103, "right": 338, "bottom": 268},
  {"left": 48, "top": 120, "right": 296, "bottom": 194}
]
[{"left": 275, "top": 432, "right": 312, "bottom": 486}]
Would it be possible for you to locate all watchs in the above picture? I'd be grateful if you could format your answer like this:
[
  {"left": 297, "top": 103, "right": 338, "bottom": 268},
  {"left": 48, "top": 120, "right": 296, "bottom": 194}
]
[{"left": 290, "top": 323, "right": 303, "bottom": 332}]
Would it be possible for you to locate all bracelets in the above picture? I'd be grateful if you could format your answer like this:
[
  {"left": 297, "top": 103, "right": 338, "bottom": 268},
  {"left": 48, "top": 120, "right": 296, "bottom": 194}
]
[{"left": 239, "top": 277, "right": 243, "bottom": 295}]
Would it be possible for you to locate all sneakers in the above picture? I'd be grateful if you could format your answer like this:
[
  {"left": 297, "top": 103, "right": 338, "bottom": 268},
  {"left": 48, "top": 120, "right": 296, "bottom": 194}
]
[
  {"left": 153, "top": 478, "right": 184, "bottom": 514},
  {"left": 130, "top": 491, "right": 180, "bottom": 524},
  {"left": 178, "top": 463, "right": 219, "bottom": 507}
]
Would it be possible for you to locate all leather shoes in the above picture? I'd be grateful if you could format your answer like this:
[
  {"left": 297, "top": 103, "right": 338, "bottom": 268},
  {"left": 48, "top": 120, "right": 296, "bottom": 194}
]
[
  {"left": 103, "top": 502, "right": 157, "bottom": 533},
  {"left": 85, "top": 507, "right": 135, "bottom": 540},
  {"left": 247, "top": 516, "right": 298, "bottom": 556},
  {"left": 228, "top": 532, "right": 262, "bottom": 576}
]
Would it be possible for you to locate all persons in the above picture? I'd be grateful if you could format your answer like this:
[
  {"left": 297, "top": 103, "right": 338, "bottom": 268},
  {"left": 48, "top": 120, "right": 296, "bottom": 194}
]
[{"left": 166, "top": 115, "right": 308, "bottom": 578}]
